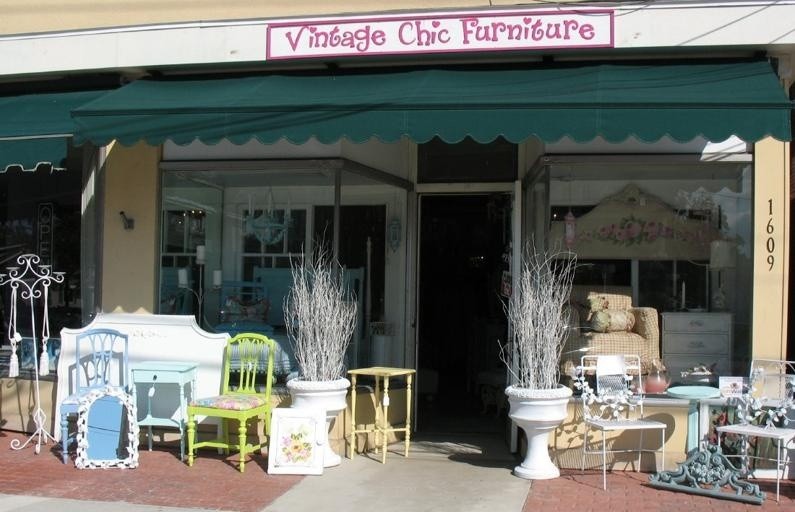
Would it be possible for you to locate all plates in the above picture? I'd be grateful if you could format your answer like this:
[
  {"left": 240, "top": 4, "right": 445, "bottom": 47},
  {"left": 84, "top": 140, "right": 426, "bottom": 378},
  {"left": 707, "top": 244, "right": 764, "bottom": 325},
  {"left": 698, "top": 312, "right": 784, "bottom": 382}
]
[{"left": 688, "top": 308, "right": 704, "bottom": 312}]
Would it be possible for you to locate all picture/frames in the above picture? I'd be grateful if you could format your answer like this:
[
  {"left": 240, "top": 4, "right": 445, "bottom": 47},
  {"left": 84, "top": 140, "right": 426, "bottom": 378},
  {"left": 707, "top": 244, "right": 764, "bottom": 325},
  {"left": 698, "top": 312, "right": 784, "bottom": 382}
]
[
  {"left": 20, "top": 338, "right": 61, "bottom": 374},
  {"left": 266, "top": 407, "right": 326, "bottom": 476}
]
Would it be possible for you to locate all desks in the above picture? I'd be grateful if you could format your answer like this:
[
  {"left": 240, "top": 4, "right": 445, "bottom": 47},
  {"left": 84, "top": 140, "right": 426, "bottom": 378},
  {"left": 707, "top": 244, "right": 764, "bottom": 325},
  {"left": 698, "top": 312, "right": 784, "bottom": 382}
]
[
  {"left": 347, "top": 366, "right": 415, "bottom": 465},
  {"left": 666, "top": 383, "right": 719, "bottom": 461},
  {"left": 132, "top": 360, "right": 197, "bottom": 461}
]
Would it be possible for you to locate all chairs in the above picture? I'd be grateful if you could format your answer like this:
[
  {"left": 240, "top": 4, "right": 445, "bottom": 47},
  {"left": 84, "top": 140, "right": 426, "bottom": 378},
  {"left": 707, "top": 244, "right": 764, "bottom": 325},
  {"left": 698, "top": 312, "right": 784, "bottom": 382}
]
[
  {"left": 554, "top": 285, "right": 663, "bottom": 393},
  {"left": 717, "top": 359, "right": 795, "bottom": 502},
  {"left": 581, "top": 354, "right": 667, "bottom": 491},
  {"left": 217, "top": 280, "right": 273, "bottom": 338},
  {"left": 187, "top": 332, "right": 274, "bottom": 473},
  {"left": 60, "top": 329, "right": 129, "bottom": 465}
]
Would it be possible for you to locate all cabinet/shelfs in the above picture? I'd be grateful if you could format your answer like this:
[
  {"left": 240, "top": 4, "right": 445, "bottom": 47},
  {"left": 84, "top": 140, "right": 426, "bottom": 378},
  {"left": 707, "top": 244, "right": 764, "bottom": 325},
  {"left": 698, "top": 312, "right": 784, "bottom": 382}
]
[{"left": 661, "top": 312, "right": 733, "bottom": 384}]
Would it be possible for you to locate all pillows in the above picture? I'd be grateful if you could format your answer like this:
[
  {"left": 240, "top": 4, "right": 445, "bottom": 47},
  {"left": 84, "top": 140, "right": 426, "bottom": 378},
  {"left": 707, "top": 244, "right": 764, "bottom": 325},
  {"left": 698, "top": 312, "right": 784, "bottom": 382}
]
[
  {"left": 221, "top": 296, "right": 268, "bottom": 324},
  {"left": 566, "top": 295, "right": 636, "bottom": 332}
]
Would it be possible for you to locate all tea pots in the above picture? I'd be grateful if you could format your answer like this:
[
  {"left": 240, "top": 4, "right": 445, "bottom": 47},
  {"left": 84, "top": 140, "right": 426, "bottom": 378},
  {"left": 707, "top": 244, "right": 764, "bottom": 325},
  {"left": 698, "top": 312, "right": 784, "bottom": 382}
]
[{"left": 643, "top": 357, "right": 671, "bottom": 394}]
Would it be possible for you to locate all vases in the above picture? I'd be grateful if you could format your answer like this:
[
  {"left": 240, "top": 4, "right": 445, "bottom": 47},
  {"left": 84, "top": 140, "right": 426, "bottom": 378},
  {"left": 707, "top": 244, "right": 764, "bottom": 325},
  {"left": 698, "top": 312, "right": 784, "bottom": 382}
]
[
  {"left": 496, "top": 385, "right": 590, "bottom": 481},
  {"left": 283, "top": 379, "right": 359, "bottom": 467}
]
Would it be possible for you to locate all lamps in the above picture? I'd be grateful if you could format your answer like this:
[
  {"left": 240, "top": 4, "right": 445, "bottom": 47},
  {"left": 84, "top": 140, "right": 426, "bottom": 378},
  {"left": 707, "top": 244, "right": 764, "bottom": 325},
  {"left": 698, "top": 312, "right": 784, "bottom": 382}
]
[
  {"left": 245, "top": 187, "right": 292, "bottom": 245},
  {"left": 710, "top": 239, "right": 736, "bottom": 310},
  {"left": 564, "top": 182, "right": 575, "bottom": 244}
]
